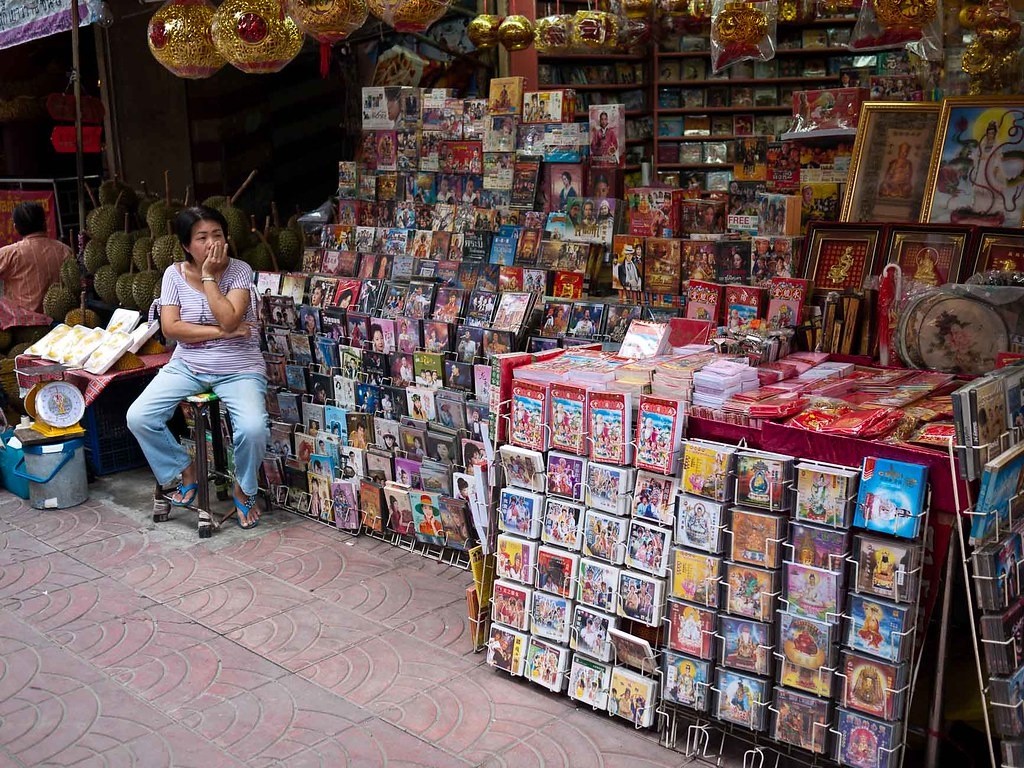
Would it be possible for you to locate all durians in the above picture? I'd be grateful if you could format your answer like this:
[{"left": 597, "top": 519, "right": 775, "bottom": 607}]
[
  {"left": 0, "top": 326, "right": 50, "bottom": 359},
  {"left": 42, "top": 179, "right": 310, "bottom": 370}
]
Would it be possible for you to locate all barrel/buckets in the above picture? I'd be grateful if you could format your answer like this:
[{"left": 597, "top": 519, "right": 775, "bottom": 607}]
[{"left": 12, "top": 438, "right": 88, "bottom": 510}]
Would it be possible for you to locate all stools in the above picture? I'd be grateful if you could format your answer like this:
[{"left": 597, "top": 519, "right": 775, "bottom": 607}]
[{"left": 152, "top": 390, "right": 273, "bottom": 539}]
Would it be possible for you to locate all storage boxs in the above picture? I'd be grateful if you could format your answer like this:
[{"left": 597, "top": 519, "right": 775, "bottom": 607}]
[
  {"left": 589, "top": 104, "right": 626, "bottom": 168},
  {"left": 684, "top": 279, "right": 727, "bottom": 325},
  {"left": 78, "top": 374, "right": 190, "bottom": 477},
  {"left": 363, "top": 86, "right": 412, "bottom": 131},
  {"left": 561, "top": 197, "right": 620, "bottom": 248},
  {"left": 754, "top": 193, "right": 803, "bottom": 236},
  {"left": 523, "top": 89, "right": 569, "bottom": 124},
  {"left": 870, "top": 75, "right": 921, "bottom": 100},
  {"left": 553, "top": 271, "right": 590, "bottom": 300},
  {"left": 627, "top": 187, "right": 683, "bottom": 238},
  {"left": 680, "top": 198, "right": 726, "bottom": 239},
  {"left": 767, "top": 276, "right": 815, "bottom": 329},
  {"left": 488, "top": 76, "right": 524, "bottom": 115},
  {"left": 545, "top": 123, "right": 590, "bottom": 162},
  {"left": 724, "top": 283, "right": 768, "bottom": 328},
  {"left": 792, "top": 88, "right": 870, "bottom": 131},
  {"left": 536, "top": 238, "right": 597, "bottom": 275}
]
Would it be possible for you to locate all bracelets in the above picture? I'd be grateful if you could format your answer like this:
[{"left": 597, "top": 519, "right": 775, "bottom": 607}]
[{"left": 201, "top": 277, "right": 216, "bottom": 283}]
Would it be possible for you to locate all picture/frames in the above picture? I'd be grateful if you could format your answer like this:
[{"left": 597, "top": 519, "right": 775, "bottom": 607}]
[
  {"left": 887, "top": 231, "right": 967, "bottom": 286},
  {"left": 919, "top": 95, "right": 1024, "bottom": 228},
  {"left": 973, "top": 233, "right": 1024, "bottom": 278},
  {"left": 804, "top": 229, "right": 880, "bottom": 298},
  {"left": 839, "top": 100, "right": 942, "bottom": 222}
]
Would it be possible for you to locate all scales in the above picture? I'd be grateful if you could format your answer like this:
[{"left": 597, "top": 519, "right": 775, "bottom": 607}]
[{"left": 13, "top": 365, "right": 89, "bottom": 437}]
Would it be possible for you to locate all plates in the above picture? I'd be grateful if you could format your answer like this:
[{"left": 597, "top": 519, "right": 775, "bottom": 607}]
[{"left": 22, "top": 307, "right": 160, "bottom": 375}]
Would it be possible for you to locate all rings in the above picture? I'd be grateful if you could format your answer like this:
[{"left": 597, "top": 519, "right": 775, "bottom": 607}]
[{"left": 214, "top": 256, "right": 218, "bottom": 258}]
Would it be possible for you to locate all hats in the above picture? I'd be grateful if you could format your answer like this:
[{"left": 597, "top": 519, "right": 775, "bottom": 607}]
[
  {"left": 593, "top": 174, "right": 608, "bottom": 185},
  {"left": 501, "top": 118, "right": 512, "bottom": 128},
  {"left": 440, "top": 401, "right": 451, "bottom": 410},
  {"left": 415, "top": 495, "right": 439, "bottom": 516},
  {"left": 623, "top": 245, "right": 634, "bottom": 254},
  {"left": 411, "top": 394, "right": 421, "bottom": 401},
  {"left": 583, "top": 199, "right": 595, "bottom": 210},
  {"left": 597, "top": 199, "right": 610, "bottom": 218},
  {"left": 382, "top": 429, "right": 396, "bottom": 441}
]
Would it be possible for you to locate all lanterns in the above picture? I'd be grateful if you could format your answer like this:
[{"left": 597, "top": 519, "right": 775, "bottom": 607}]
[
  {"left": 147, "top": 0, "right": 453, "bottom": 80},
  {"left": 472, "top": 1, "right": 938, "bottom": 72}
]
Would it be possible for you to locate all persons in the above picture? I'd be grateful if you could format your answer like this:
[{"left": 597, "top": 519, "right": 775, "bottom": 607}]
[
  {"left": 399, "top": 354, "right": 483, "bottom": 441},
  {"left": 487, "top": 454, "right": 662, "bottom": 721},
  {"left": 397, "top": 322, "right": 414, "bottom": 353},
  {"left": 384, "top": 430, "right": 480, "bottom": 499},
  {"left": 415, "top": 495, "right": 442, "bottom": 536},
  {"left": 439, "top": 291, "right": 630, "bottom": 363},
  {"left": 306, "top": 285, "right": 385, "bottom": 352},
  {"left": 613, "top": 191, "right": 671, "bottom": 289},
  {"left": 325, "top": 85, "right": 618, "bottom": 252},
  {"left": 274, "top": 441, "right": 288, "bottom": 454},
  {"left": 687, "top": 141, "right": 821, "bottom": 281},
  {"left": 429, "top": 328, "right": 446, "bottom": 348},
  {"left": 0, "top": 202, "right": 74, "bottom": 330},
  {"left": 276, "top": 312, "right": 288, "bottom": 325},
  {"left": 126, "top": 206, "right": 270, "bottom": 530},
  {"left": 384, "top": 286, "right": 431, "bottom": 315},
  {"left": 310, "top": 382, "right": 391, "bottom": 515},
  {"left": 390, "top": 496, "right": 407, "bottom": 534}
]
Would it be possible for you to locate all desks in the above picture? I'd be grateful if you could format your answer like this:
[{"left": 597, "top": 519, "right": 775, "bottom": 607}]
[
  {"left": 483, "top": 342, "right": 984, "bottom": 768},
  {"left": 15, "top": 351, "right": 172, "bottom": 482}
]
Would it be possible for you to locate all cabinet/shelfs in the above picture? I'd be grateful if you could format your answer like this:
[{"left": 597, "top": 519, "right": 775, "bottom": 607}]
[{"left": 506, "top": 0, "right": 953, "bottom": 189}]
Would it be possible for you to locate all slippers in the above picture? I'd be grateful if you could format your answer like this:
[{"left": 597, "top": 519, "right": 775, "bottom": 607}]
[
  {"left": 232, "top": 484, "right": 260, "bottom": 529},
  {"left": 172, "top": 481, "right": 198, "bottom": 506}
]
